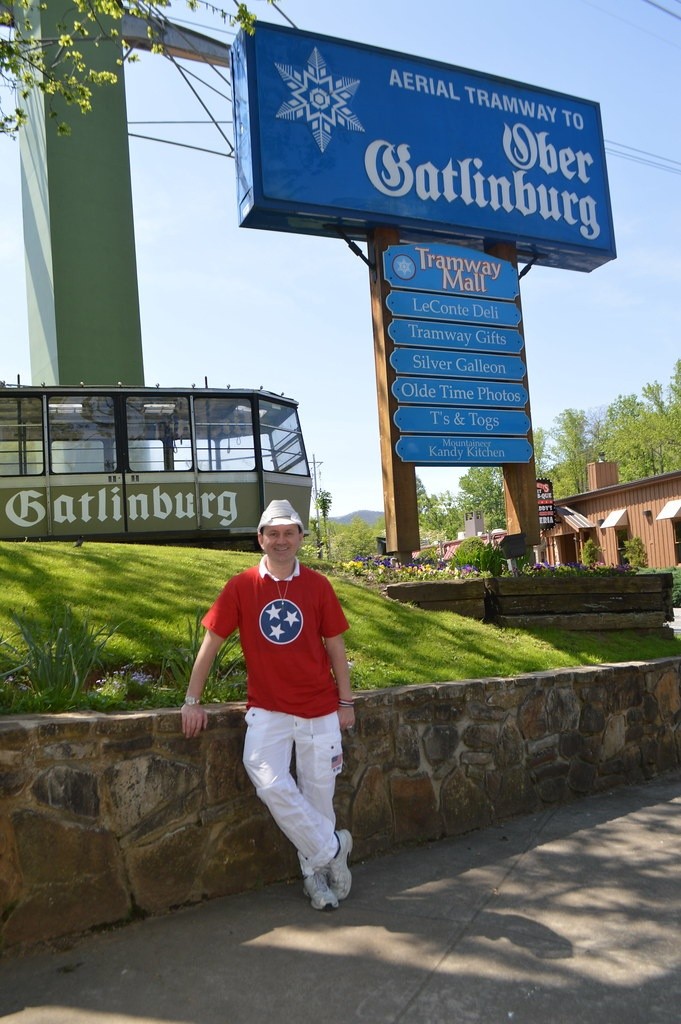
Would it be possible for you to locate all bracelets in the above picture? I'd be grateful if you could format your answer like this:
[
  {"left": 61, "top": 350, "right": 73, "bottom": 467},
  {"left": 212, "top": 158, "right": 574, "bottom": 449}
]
[{"left": 338, "top": 699, "right": 354, "bottom": 707}]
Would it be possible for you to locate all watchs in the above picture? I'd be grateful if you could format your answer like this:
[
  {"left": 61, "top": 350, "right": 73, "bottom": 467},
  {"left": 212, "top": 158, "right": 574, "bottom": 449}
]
[{"left": 183, "top": 696, "right": 200, "bottom": 706}]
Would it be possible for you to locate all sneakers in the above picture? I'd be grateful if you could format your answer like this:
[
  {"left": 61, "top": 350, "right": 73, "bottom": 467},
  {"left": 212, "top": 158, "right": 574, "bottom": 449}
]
[
  {"left": 303, "top": 871, "right": 339, "bottom": 912},
  {"left": 328, "top": 829, "right": 353, "bottom": 900}
]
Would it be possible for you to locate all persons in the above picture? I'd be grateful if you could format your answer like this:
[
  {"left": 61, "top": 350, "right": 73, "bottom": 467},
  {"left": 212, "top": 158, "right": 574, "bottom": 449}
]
[{"left": 180, "top": 500, "right": 356, "bottom": 912}]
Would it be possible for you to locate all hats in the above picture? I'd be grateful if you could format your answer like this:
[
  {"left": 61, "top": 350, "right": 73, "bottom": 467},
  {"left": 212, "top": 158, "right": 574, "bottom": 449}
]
[{"left": 257, "top": 499, "right": 305, "bottom": 550}]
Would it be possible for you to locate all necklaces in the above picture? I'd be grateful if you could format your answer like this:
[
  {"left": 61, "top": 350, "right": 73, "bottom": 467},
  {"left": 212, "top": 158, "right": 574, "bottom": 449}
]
[{"left": 265, "top": 563, "right": 295, "bottom": 605}]
